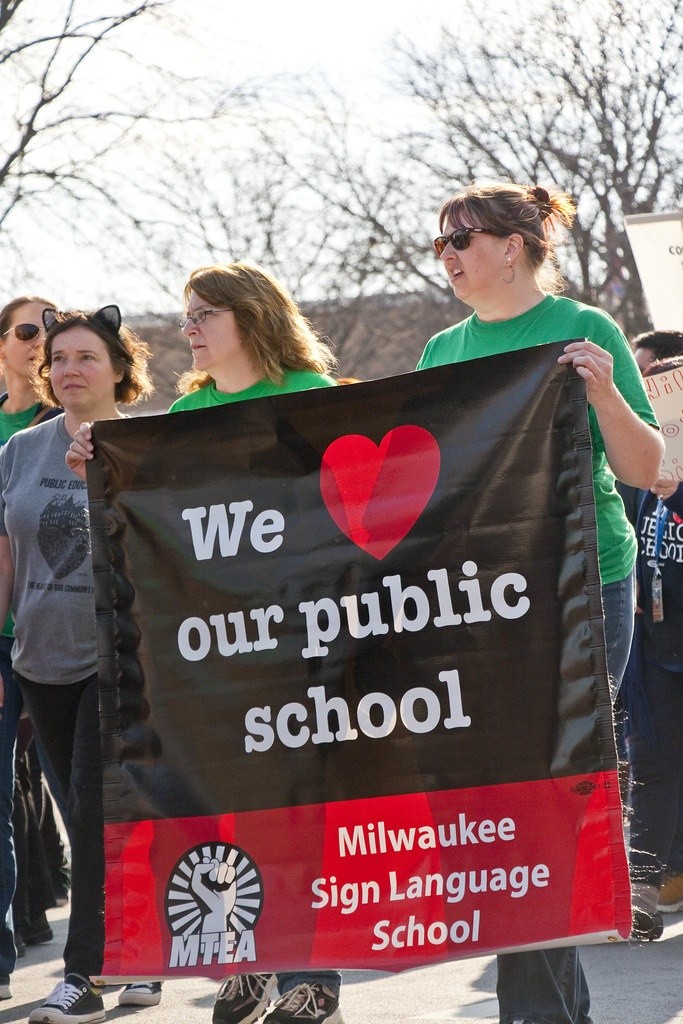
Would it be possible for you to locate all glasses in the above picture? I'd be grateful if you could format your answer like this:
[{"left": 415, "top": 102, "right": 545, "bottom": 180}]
[
  {"left": 3, "top": 323, "right": 46, "bottom": 342},
  {"left": 178, "top": 309, "right": 234, "bottom": 331},
  {"left": 434, "top": 228, "right": 530, "bottom": 256}
]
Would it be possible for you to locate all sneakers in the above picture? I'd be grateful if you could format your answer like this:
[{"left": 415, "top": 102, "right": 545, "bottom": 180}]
[
  {"left": 262, "top": 982, "right": 344, "bottom": 1024},
  {"left": 29, "top": 972, "right": 106, "bottom": 1024},
  {"left": 119, "top": 980, "right": 162, "bottom": 1005},
  {"left": 212, "top": 972, "right": 278, "bottom": 1024}
]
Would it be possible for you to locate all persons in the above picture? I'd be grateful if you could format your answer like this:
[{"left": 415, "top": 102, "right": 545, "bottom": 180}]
[
  {"left": 0, "top": 266, "right": 368, "bottom": 1024},
  {"left": 409, "top": 183, "right": 683, "bottom": 1024}
]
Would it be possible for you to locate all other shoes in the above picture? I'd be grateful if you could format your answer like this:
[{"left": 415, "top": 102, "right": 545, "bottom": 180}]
[
  {"left": 21, "top": 914, "right": 52, "bottom": 944},
  {"left": 13, "top": 933, "right": 26, "bottom": 956},
  {"left": 656, "top": 872, "right": 683, "bottom": 913},
  {"left": 630, "top": 909, "right": 664, "bottom": 942},
  {"left": 54, "top": 893, "right": 68, "bottom": 907},
  {"left": 0, "top": 975, "right": 11, "bottom": 999}
]
